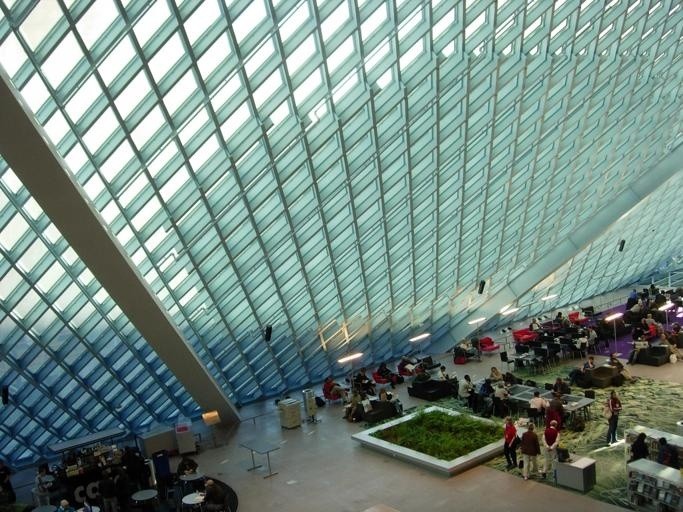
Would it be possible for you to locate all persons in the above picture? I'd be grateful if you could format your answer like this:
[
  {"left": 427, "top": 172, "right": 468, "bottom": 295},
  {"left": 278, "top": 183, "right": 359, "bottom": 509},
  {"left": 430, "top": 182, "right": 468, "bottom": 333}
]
[
  {"left": 176, "top": 456, "right": 198, "bottom": 475},
  {"left": 200, "top": 479, "right": 227, "bottom": 511},
  {"left": 35, "top": 469, "right": 54, "bottom": 484},
  {"left": 118, "top": 446, "right": 142, "bottom": 475},
  {"left": 0, "top": 461, "right": 17, "bottom": 504},
  {"left": 100, "top": 465, "right": 119, "bottom": 511},
  {"left": 57, "top": 500, "right": 77, "bottom": 512}
]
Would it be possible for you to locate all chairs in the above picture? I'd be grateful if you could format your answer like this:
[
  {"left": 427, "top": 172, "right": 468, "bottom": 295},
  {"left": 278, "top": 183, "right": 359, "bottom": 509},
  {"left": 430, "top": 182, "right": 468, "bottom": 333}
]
[
  {"left": 620, "top": 284, "right": 683, "bottom": 366},
  {"left": 219, "top": 494, "right": 231, "bottom": 512},
  {"left": 500, "top": 304, "right": 625, "bottom": 388},
  {"left": 556, "top": 447, "right": 570, "bottom": 462},
  {"left": 461, "top": 372, "right": 597, "bottom": 423},
  {"left": 322, "top": 383, "right": 342, "bottom": 404},
  {"left": 397, "top": 357, "right": 459, "bottom": 400},
  {"left": 345, "top": 369, "right": 403, "bottom": 421},
  {"left": 453, "top": 336, "right": 500, "bottom": 365}
]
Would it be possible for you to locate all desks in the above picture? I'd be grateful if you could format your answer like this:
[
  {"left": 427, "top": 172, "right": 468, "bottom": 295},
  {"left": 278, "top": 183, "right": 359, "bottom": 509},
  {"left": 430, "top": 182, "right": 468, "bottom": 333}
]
[
  {"left": 30, "top": 449, "right": 207, "bottom": 512},
  {"left": 240, "top": 437, "right": 280, "bottom": 479},
  {"left": 48, "top": 426, "right": 127, "bottom": 458}
]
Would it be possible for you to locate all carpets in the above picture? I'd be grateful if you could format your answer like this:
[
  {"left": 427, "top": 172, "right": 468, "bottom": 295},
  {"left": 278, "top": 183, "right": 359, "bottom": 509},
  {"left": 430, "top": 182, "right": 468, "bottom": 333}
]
[{"left": 22, "top": 473, "right": 239, "bottom": 512}]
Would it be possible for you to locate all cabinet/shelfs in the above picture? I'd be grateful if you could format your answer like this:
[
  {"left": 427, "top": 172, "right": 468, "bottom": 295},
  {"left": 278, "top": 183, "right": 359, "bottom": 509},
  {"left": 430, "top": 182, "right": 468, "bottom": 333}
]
[
  {"left": 278, "top": 399, "right": 301, "bottom": 428},
  {"left": 624, "top": 420, "right": 683, "bottom": 512},
  {"left": 553, "top": 455, "right": 597, "bottom": 492},
  {"left": 139, "top": 426, "right": 175, "bottom": 457}
]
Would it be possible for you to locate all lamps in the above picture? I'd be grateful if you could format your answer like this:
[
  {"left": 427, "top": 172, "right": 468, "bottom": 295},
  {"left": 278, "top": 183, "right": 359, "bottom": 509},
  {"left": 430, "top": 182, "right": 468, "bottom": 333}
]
[
  {"left": 2, "top": 384, "right": 10, "bottom": 404},
  {"left": 619, "top": 240, "right": 625, "bottom": 251},
  {"left": 478, "top": 279, "right": 485, "bottom": 295},
  {"left": 264, "top": 324, "right": 273, "bottom": 341},
  {"left": 201, "top": 410, "right": 222, "bottom": 450}
]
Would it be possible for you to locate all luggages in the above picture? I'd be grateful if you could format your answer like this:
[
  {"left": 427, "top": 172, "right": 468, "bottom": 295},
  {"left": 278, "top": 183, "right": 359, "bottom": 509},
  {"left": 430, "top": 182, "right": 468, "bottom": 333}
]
[{"left": 315, "top": 395, "right": 326, "bottom": 407}]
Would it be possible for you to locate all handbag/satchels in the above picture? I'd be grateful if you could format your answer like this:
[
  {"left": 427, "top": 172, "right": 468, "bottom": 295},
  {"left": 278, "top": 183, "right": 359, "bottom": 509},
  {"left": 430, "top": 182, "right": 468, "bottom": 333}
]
[
  {"left": 511, "top": 437, "right": 522, "bottom": 450},
  {"left": 518, "top": 458, "right": 524, "bottom": 469},
  {"left": 602, "top": 407, "right": 613, "bottom": 419}
]
